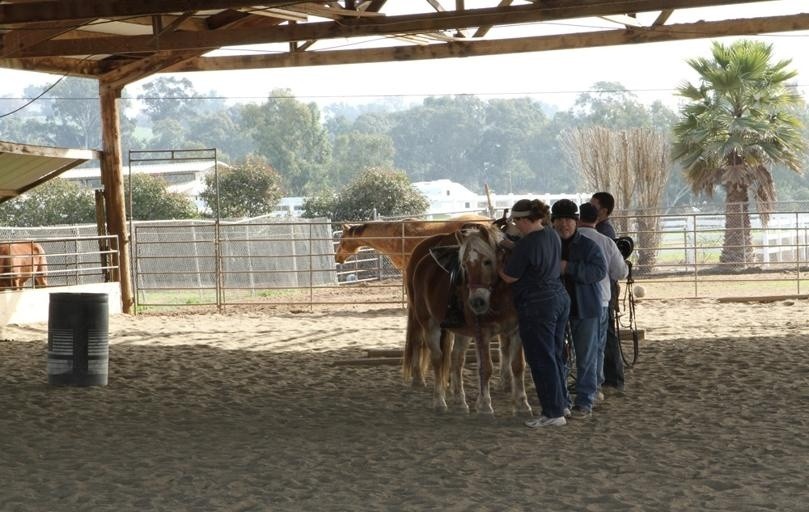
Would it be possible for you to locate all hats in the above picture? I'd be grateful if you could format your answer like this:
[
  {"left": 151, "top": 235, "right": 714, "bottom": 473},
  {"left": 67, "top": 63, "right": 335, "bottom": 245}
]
[
  {"left": 506, "top": 210, "right": 531, "bottom": 224},
  {"left": 551, "top": 199, "right": 579, "bottom": 222}
]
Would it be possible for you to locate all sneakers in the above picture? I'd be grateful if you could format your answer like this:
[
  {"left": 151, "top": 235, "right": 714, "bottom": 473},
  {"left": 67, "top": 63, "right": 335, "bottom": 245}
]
[
  {"left": 524, "top": 414, "right": 568, "bottom": 428},
  {"left": 597, "top": 384, "right": 621, "bottom": 401},
  {"left": 570, "top": 403, "right": 593, "bottom": 420}
]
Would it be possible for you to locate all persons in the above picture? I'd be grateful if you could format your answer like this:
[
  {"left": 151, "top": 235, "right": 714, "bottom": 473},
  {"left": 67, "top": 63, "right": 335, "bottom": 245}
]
[
  {"left": 551, "top": 198, "right": 607, "bottom": 419},
  {"left": 590, "top": 191, "right": 624, "bottom": 388},
  {"left": 499, "top": 200, "right": 571, "bottom": 428},
  {"left": 577, "top": 203, "right": 629, "bottom": 398}
]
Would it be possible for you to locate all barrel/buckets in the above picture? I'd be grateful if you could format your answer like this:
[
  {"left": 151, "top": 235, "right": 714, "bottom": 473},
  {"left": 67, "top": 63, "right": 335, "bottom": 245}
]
[{"left": 48, "top": 292, "right": 109, "bottom": 387}]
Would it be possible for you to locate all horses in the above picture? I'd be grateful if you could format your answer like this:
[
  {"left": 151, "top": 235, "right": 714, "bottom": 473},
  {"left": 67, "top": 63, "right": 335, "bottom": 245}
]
[
  {"left": 403, "top": 222, "right": 534, "bottom": 423},
  {"left": 334, "top": 215, "right": 493, "bottom": 272},
  {"left": 0, "top": 241, "right": 48, "bottom": 291}
]
